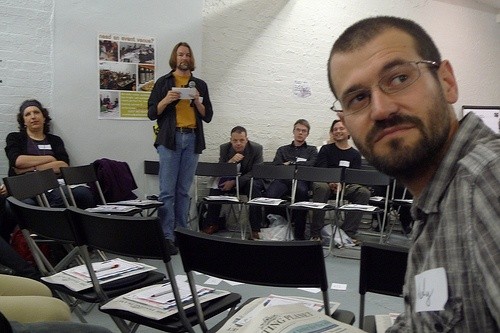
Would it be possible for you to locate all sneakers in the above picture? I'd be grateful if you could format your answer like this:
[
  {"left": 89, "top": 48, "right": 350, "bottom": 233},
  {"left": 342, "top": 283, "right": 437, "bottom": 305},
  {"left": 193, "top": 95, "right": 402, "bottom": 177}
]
[
  {"left": 354, "top": 237, "right": 359, "bottom": 245},
  {"left": 311, "top": 237, "right": 321, "bottom": 241}
]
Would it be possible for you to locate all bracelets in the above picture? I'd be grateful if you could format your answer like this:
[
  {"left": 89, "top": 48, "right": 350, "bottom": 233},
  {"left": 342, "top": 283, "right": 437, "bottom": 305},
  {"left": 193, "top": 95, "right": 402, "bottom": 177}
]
[{"left": 32, "top": 165, "right": 37, "bottom": 172}]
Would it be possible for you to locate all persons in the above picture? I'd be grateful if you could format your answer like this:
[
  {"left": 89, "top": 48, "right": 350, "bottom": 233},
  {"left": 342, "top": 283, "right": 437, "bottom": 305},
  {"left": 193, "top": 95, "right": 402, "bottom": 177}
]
[
  {"left": 327, "top": 18, "right": 500, "bottom": 333},
  {"left": 308, "top": 119, "right": 371, "bottom": 245},
  {"left": 263, "top": 119, "right": 317, "bottom": 241},
  {"left": 203, "top": 126, "right": 263, "bottom": 239},
  {"left": 0, "top": 184, "right": 112, "bottom": 333},
  {"left": 4, "top": 99, "right": 97, "bottom": 259},
  {"left": 147, "top": 42, "right": 213, "bottom": 255}
]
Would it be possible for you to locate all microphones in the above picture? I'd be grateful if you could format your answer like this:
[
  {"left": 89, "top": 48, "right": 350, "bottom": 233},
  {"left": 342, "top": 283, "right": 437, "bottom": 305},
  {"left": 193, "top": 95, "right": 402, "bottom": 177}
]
[{"left": 189, "top": 81, "right": 196, "bottom": 107}]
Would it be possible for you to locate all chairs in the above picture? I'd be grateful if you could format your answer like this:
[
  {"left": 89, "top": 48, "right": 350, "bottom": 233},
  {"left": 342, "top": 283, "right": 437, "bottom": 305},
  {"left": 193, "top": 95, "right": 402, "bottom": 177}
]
[{"left": 2, "top": 158, "right": 412, "bottom": 333}]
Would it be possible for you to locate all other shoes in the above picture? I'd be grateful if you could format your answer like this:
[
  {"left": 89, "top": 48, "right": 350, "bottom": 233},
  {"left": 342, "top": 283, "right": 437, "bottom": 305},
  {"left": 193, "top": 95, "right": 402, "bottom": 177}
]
[{"left": 166, "top": 239, "right": 178, "bottom": 256}]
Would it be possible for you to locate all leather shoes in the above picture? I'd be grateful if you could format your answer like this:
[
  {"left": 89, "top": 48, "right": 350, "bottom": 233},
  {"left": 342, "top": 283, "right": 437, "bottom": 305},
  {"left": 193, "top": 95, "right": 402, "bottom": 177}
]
[
  {"left": 248, "top": 232, "right": 260, "bottom": 240},
  {"left": 203, "top": 225, "right": 218, "bottom": 234}
]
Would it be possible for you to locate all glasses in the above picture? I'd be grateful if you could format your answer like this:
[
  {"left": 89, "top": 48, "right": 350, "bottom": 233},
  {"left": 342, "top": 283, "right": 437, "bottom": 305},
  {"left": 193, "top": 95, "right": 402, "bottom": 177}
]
[{"left": 329, "top": 60, "right": 439, "bottom": 114}]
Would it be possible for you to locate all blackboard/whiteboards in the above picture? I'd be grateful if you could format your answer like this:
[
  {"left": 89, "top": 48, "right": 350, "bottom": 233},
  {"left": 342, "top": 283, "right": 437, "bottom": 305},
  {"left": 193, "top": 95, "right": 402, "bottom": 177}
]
[{"left": 462, "top": 105, "right": 500, "bottom": 134}]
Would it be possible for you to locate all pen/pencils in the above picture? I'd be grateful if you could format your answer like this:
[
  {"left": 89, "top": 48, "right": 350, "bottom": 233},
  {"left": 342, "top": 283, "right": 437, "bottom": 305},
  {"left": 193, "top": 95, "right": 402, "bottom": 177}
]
[
  {"left": 152, "top": 289, "right": 172, "bottom": 298},
  {"left": 94, "top": 263, "right": 119, "bottom": 272},
  {"left": 130, "top": 200, "right": 142, "bottom": 202},
  {"left": 97, "top": 205, "right": 117, "bottom": 210}
]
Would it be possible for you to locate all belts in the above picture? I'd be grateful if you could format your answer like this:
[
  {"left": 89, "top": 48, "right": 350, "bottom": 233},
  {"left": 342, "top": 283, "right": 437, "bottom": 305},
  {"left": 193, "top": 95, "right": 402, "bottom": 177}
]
[{"left": 176, "top": 127, "right": 197, "bottom": 133}]
